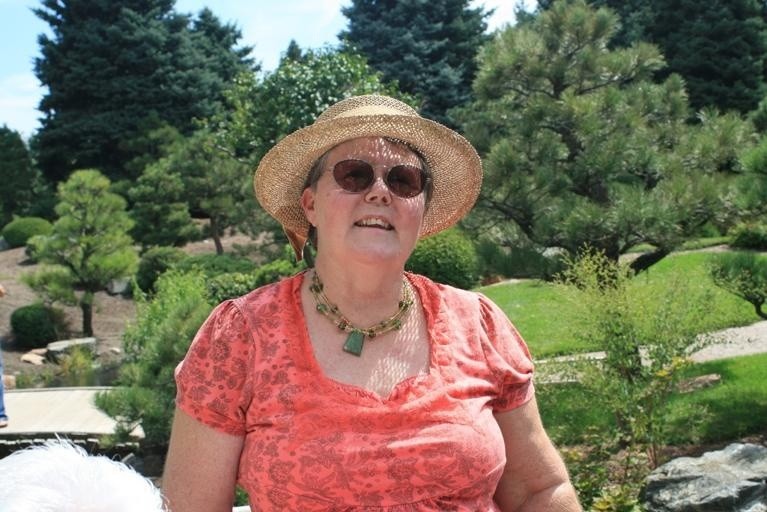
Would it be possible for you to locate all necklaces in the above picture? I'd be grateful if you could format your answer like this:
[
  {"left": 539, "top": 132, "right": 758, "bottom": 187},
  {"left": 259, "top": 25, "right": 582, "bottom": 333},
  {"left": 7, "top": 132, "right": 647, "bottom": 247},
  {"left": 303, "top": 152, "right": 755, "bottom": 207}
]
[{"left": 304, "top": 264, "right": 416, "bottom": 359}]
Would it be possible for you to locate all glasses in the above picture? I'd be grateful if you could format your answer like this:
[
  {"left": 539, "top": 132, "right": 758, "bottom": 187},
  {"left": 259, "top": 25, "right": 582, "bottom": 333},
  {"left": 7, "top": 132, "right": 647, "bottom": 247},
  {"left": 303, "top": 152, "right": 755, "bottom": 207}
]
[{"left": 317, "top": 158, "right": 433, "bottom": 201}]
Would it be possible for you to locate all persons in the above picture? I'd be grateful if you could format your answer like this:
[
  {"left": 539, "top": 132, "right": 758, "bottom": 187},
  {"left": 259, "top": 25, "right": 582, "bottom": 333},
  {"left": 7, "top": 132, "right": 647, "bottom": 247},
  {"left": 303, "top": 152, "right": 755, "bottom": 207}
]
[{"left": 157, "top": 94, "right": 584, "bottom": 512}]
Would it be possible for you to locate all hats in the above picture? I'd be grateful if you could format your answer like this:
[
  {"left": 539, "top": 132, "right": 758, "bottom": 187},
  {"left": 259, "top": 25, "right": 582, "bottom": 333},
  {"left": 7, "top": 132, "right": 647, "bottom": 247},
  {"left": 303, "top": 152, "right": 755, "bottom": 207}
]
[{"left": 251, "top": 92, "right": 484, "bottom": 243}]
